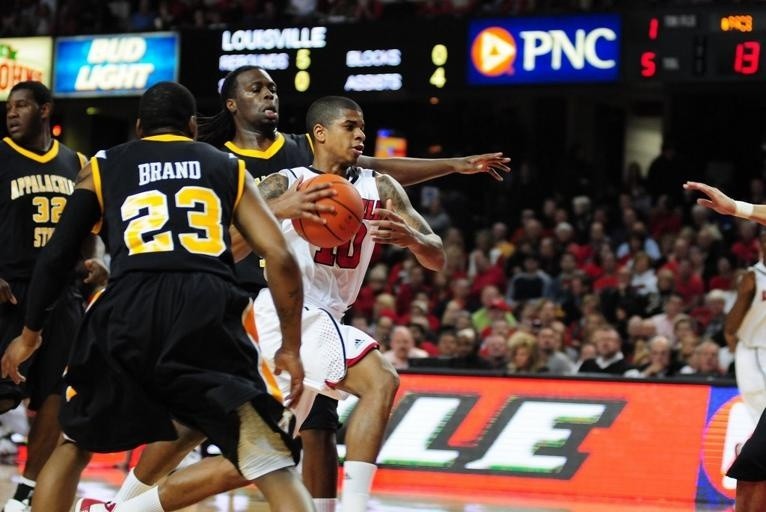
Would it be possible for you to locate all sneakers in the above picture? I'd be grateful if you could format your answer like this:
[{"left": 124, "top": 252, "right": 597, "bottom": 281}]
[
  {"left": 1, "top": 499, "right": 31, "bottom": 512},
  {"left": 75, "top": 497, "right": 117, "bottom": 512}
]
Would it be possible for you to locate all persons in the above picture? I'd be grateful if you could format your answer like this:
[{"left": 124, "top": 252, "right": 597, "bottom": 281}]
[{"left": 0, "top": 0, "right": 766, "bottom": 512}]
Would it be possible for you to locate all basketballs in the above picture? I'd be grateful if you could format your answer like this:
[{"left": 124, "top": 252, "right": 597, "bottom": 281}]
[{"left": 291, "top": 174, "right": 364, "bottom": 248}]
